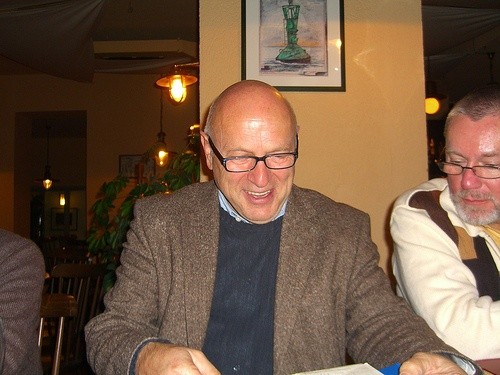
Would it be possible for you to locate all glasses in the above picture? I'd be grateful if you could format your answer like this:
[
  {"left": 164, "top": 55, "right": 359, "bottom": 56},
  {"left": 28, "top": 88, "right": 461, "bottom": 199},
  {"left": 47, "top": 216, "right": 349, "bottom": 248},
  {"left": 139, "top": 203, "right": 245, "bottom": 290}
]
[
  {"left": 437, "top": 145, "right": 500, "bottom": 179},
  {"left": 204, "top": 133, "right": 299, "bottom": 173}
]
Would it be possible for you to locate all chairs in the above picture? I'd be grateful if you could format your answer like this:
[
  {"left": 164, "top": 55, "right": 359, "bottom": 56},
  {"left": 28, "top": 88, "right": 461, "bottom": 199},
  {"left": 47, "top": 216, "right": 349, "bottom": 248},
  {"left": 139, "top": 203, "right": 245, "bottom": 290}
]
[{"left": 28, "top": 237, "right": 117, "bottom": 375}]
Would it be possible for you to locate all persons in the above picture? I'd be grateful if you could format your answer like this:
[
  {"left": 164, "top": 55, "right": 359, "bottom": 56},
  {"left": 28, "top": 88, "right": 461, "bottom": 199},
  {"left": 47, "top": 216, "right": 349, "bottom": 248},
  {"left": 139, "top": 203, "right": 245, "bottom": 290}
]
[
  {"left": 390, "top": 86, "right": 500, "bottom": 375},
  {"left": 0, "top": 228, "right": 45, "bottom": 375},
  {"left": 85, "top": 80, "right": 485, "bottom": 375}
]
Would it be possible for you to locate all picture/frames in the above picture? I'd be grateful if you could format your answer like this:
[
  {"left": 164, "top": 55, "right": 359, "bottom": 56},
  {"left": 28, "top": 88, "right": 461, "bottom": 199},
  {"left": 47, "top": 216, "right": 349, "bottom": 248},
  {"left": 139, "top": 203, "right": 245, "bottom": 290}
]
[
  {"left": 50, "top": 207, "right": 79, "bottom": 230},
  {"left": 119, "top": 154, "right": 156, "bottom": 178},
  {"left": 242, "top": 0, "right": 346, "bottom": 92}
]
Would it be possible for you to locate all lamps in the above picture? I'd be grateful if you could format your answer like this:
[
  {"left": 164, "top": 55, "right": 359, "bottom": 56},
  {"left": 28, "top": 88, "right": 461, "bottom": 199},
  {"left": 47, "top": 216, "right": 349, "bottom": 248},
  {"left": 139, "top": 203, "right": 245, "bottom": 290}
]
[
  {"left": 41, "top": 118, "right": 54, "bottom": 190},
  {"left": 152, "top": 67, "right": 168, "bottom": 168},
  {"left": 155, "top": 61, "right": 201, "bottom": 104}
]
